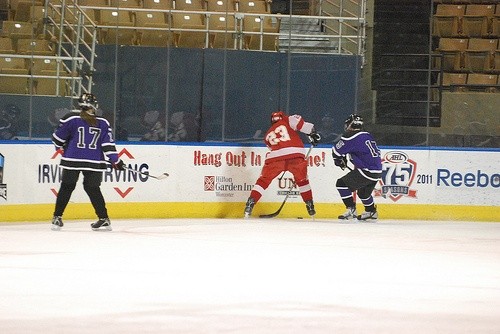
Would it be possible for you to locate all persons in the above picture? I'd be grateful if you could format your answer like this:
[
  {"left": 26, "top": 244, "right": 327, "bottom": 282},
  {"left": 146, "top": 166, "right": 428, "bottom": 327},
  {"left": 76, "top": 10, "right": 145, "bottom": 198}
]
[
  {"left": 51, "top": 93, "right": 126, "bottom": 231},
  {"left": 244, "top": 111, "right": 321, "bottom": 220},
  {"left": 0, "top": 104, "right": 19, "bottom": 140},
  {"left": 332, "top": 114, "right": 382, "bottom": 223},
  {"left": 139, "top": 110, "right": 206, "bottom": 142}
]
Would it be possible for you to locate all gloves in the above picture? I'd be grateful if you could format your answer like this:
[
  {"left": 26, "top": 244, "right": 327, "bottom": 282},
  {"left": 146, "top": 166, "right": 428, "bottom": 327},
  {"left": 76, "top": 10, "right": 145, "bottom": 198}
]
[
  {"left": 333, "top": 152, "right": 348, "bottom": 169},
  {"left": 306, "top": 127, "right": 321, "bottom": 147},
  {"left": 115, "top": 158, "right": 125, "bottom": 170}
]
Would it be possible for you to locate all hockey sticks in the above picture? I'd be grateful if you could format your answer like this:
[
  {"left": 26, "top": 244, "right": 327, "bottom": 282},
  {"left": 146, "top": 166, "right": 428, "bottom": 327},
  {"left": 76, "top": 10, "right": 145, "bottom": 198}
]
[
  {"left": 337, "top": 166, "right": 356, "bottom": 219},
  {"left": 258, "top": 145, "right": 313, "bottom": 219},
  {"left": 122, "top": 165, "right": 170, "bottom": 180}
]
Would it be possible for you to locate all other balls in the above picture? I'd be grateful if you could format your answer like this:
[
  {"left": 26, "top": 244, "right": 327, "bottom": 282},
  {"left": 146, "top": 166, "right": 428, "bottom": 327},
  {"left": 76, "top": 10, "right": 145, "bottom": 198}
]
[{"left": 298, "top": 217, "right": 303, "bottom": 219}]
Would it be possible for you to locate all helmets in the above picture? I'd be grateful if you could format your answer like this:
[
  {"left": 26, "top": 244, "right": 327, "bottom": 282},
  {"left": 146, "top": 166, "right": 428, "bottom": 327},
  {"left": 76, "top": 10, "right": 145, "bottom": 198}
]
[
  {"left": 0, "top": 104, "right": 20, "bottom": 132},
  {"left": 345, "top": 113, "right": 364, "bottom": 131},
  {"left": 271, "top": 111, "right": 284, "bottom": 124},
  {"left": 78, "top": 93, "right": 98, "bottom": 110}
]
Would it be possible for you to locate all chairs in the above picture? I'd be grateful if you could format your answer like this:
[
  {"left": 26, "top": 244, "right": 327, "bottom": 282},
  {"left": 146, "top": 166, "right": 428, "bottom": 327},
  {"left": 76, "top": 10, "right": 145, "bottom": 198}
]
[
  {"left": 1, "top": 0, "right": 281, "bottom": 96},
  {"left": 429, "top": 3, "right": 500, "bottom": 105}
]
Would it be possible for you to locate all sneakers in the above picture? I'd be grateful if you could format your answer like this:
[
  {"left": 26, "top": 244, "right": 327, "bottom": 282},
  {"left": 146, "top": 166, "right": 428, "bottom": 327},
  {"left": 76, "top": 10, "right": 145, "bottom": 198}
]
[
  {"left": 244, "top": 197, "right": 254, "bottom": 217},
  {"left": 52, "top": 216, "right": 64, "bottom": 230},
  {"left": 338, "top": 202, "right": 359, "bottom": 223},
  {"left": 91, "top": 218, "right": 112, "bottom": 232},
  {"left": 306, "top": 201, "right": 316, "bottom": 221},
  {"left": 357, "top": 203, "right": 378, "bottom": 223}
]
